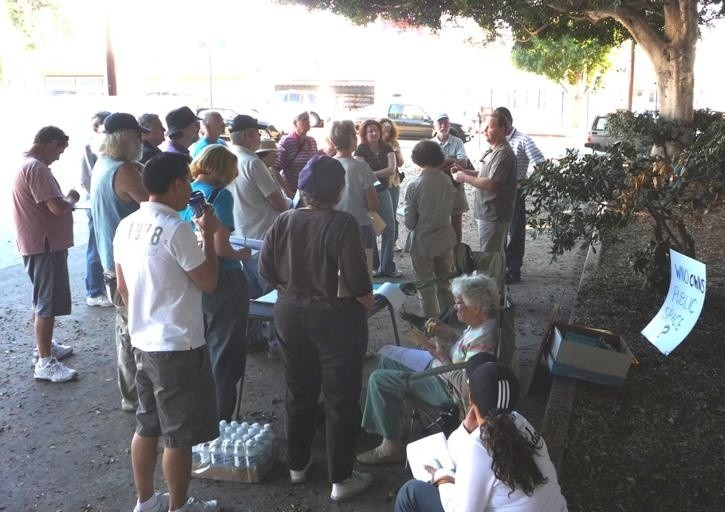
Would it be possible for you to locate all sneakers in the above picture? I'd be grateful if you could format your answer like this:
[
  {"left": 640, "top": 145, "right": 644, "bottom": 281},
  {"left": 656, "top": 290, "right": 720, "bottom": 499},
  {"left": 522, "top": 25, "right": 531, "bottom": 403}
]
[
  {"left": 330, "top": 470, "right": 373, "bottom": 501},
  {"left": 393, "top": 245, "right": 402, "bottom": 252},
  {"left": 289, "top": 456, "right": 312, "bottom": 483},
  {"left": 357, "top": 448, "right": 406, "bottom": 464},
  {"left": 372, "top": 269, "right": 403, "bottom": 278},
  {"left": 168, "top": 497, "right": 221, "bottom": 512},
  {"left": 247, "top": 335, "right": 280, "bottom": 359},
  {"left": 133, "top": 492, "right": 171, "bottom": 512},
  {"left": 32, "top": 340, "right": 72, "bottom": 364},
  {"left": 86, "top": 292, "right": 114, "bottom": 307},
  {"left": 34, "top": 358, "right": 78, "bottom": 383},
  {"left": 506, "top": 271, "right": 520, "bottom": 284}
]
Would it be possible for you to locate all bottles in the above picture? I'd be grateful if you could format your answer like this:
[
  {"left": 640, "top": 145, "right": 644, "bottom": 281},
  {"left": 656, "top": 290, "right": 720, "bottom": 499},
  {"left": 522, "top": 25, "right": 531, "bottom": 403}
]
[{"left": 192, "top": 417, "right": 275, "bottom": 467}]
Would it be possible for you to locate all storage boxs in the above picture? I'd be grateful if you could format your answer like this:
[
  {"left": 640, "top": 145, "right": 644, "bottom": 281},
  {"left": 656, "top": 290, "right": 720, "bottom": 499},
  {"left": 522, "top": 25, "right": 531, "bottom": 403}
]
[{"left": 543, "top": 322, "right": 640, "bottom": 389}]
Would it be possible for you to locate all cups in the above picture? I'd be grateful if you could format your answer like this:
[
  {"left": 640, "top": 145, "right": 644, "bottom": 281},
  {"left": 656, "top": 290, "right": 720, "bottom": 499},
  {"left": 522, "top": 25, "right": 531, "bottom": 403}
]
[{"left": 188, "top": 191, "right": 208, "bottom": 217}]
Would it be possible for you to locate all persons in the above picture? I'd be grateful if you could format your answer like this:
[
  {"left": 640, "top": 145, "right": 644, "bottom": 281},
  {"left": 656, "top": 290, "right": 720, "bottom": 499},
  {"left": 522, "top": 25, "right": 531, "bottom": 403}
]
[
  {"left": 88, "top": 111, "right": 157, "bottom": 411},
  {"left": 256, "top": 152, "right": 383, "bottom": 501},
  {"left": 112, "top": 149, "right": 224, "bottom": 512},
  {"left": 391, "top": 349, "right": 569, "bottom": 511},
  {"left": 174, "top": 142, "right": 251, "bottom": 421},
  {"left": 352, "top": 272, "right": 502, "bottom": 465},
  {"left": 226, "top": 109, "right": 293, "bottom": 359},
  {"left": 11, "top": 125, "right": 81, "bottom": 386},
  {"left": 73, "top": 102, "right": 548, "bottom": 340}
]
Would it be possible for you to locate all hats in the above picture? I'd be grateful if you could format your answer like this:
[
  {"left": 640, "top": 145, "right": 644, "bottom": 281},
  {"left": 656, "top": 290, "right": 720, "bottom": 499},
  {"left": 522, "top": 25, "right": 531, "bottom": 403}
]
[
  {"left": 104, "top": 113, "right": 152, "bottom": 134},
  {"left": 465, "top": 351, "right": 518, "bottom": 413},
  {"left": 166, "top": 106, "right": 202, "bottom": 137},
  {"left": 297, "top": 155, "right": 345, "bottom": 196},
  {"left": 434, "top": 112, "right": 448, "bottom": 121},
  {"left": 228, "top": 114, "right": 267, "bottom": 132},
  {"left": 254, "top": 140, "right": 286, "bottom": 154}
]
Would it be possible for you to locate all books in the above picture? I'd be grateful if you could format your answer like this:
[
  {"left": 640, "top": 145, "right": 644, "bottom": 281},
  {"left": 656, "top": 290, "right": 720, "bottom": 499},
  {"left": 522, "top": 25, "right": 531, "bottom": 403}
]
[{"left": 405, "top": 431, "right": 455, "bottom": 483}]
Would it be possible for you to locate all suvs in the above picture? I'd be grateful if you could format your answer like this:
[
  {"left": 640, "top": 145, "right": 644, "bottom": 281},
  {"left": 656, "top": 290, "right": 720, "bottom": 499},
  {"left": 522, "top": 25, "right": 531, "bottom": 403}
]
[{"left": 583, "top": 114, "right": 632, "bottom": 153}]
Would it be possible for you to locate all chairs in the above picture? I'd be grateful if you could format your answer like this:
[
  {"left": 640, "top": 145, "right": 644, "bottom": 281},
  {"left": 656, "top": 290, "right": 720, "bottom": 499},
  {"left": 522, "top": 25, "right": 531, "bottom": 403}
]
[{"left": 388, "top": 244, "right": 515, "bottom": 472}]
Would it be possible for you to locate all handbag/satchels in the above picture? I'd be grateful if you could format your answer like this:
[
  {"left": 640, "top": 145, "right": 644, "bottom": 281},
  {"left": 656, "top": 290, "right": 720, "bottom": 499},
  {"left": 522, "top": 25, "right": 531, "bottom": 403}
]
[
  {"left": 241, "top": 252, "right": 276, "bottom": 299},
  {"left": 422, "top": 403, "right": 460, "bottom": 439}
]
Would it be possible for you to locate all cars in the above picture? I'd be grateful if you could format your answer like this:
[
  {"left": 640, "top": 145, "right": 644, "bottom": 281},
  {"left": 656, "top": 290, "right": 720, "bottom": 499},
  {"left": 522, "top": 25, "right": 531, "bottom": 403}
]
[
  {"left": 349, "top": 99, "right": 477, "bottom": 145},
  {"left": 46, "top": 81, "right": 333, "bottom": 142}
]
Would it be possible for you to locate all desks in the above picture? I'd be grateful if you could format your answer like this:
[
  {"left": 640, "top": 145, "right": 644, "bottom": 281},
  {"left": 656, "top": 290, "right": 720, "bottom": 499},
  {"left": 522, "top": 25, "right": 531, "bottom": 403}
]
[{"left": 235, "top": 283, "right": 422, "bottom": 425}]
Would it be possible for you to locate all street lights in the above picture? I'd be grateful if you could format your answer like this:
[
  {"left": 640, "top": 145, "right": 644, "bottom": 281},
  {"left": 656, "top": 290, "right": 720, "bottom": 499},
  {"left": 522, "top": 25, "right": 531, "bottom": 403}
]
[{"left": 198, "top": 41, "right": 214, "bottom": 111}]
